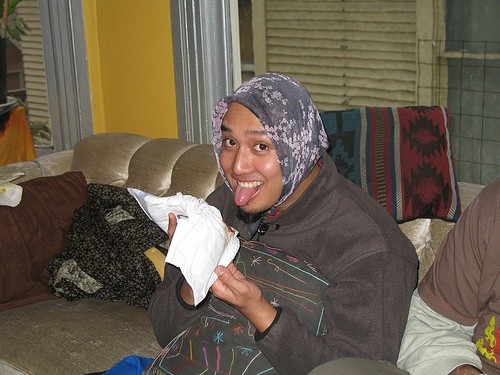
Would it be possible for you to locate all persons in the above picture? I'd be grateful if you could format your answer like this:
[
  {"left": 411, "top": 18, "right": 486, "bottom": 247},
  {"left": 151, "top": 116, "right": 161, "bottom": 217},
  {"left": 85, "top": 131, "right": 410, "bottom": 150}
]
[
  {"left": 309, "top": 177, "right": 500, "bottom": 375},
  {"left": 142, "top": 73, "right": 419, "bottom": 375}
]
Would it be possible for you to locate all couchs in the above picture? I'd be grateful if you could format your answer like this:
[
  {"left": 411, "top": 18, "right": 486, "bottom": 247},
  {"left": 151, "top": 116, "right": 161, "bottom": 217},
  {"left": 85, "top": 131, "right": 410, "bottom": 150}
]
[{"left": 0, "top": 130, "right": 484, "bottom": 375}]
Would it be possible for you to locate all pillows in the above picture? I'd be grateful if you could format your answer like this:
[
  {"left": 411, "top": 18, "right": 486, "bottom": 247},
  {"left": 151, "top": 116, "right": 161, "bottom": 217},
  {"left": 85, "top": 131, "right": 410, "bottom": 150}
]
[
  {"left": 142, "top": 239, "right": 332, "bottom": 375},
  {"left": 319, "top": 104, "right": 460, "bottom": 223},
  {"left": 0, "top": 171, "right": 90, "bottom": 311}
]
